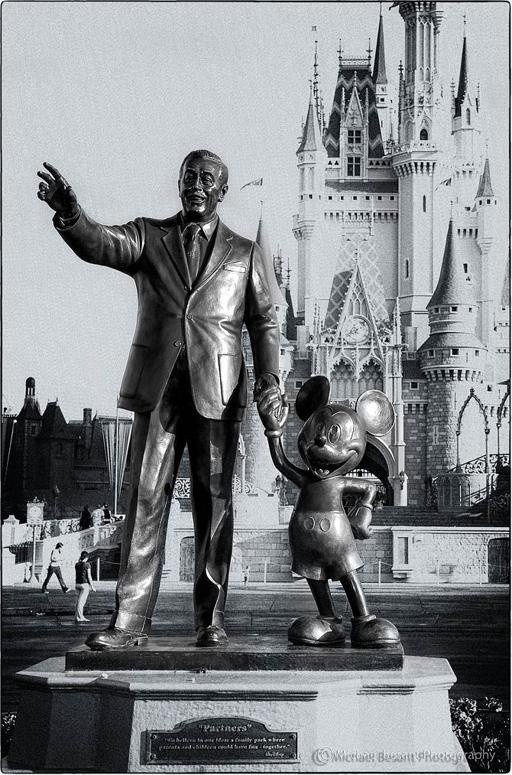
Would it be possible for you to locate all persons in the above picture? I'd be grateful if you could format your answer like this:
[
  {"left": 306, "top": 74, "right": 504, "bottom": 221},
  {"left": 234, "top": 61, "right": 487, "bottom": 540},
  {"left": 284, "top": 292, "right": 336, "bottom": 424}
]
[
  {"left": 29, "top": 146, "right": 283, "bottom": 653},
  {"left": 102, "top": 505, "right": 112, "bottom": 524},
  {"left": 41, "top": 542, "right": 72, "bottom": 594},
  {"left": 80, "top": 505, "right": 91, "bottom": 532},
  {"left": 89, "top": 504, "right": 105, "bottom": 530},
  {"left": 74, "top": 551, "right": 97, "bottom": 623},
  {"left": 243, "top": 565, "right": 251, "bottom": 588}
]
